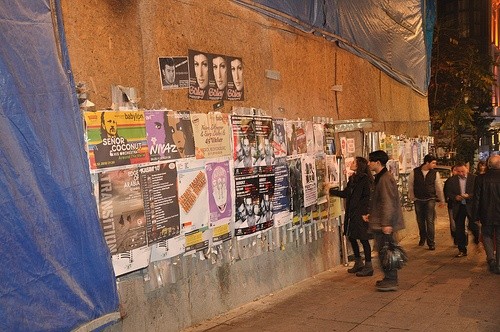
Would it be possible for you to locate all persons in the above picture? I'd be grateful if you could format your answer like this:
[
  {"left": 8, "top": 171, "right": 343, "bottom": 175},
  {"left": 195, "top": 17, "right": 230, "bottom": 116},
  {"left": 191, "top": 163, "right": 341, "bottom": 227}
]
[
  {"left": 329, "top": 157, "right": 375, "bottom": 277},
  {"left": 409, "top": 154, "right": 444, "bottom": 250},
  {"left": 234, "top": 136, "right": 256, "bottom": 168},
  {"left": 443, "top": 154, "right": 500, "bottom": 274},
  {"left": 255, "top": 138, "right": 274, "bottom": 166},
  {"left": 189, "top": 52, "right": 244, "bottom": 101},
  {"left": 367, "top": 150, "right": 405, "bottom": 291},
  {"left": 160, "top": 57, "right": 179, "bottom": 86},
  {"left": 235, "top": 194, "right": 273, "bottom": 229}
]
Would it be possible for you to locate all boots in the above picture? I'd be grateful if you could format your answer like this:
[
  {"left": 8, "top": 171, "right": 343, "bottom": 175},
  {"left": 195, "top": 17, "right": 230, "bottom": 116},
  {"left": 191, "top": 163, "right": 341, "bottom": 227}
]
[
  {"left": 348, "top": 258, "right": 364, "bottom": 273},
  {"left": 357, "top": 261, "right": 373, "bottom": 276}
]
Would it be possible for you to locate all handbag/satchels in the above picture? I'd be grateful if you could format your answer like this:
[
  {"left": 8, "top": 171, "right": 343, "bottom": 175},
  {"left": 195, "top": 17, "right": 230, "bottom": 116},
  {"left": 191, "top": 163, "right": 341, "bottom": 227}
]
[{"left": 380, "top": 233, "right": 409, "bottom": 272}]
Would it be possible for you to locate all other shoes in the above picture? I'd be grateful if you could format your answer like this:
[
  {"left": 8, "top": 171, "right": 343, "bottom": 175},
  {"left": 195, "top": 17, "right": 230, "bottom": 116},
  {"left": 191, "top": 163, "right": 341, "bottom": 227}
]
[
  {"left": 457, "top": 251, "right": 467, "bottom": 257},
  {"left": 418, "top": 240, "right": 425, "bottom": 246},
  {"left": 489, "top": 265, "right": 500, "bottom": 274},
  {"left": 428, "top": 245, "right": 435, "bottom": 250},
  {"left": 375, "top": 279, "right": 399, "bottom": 290}
]
